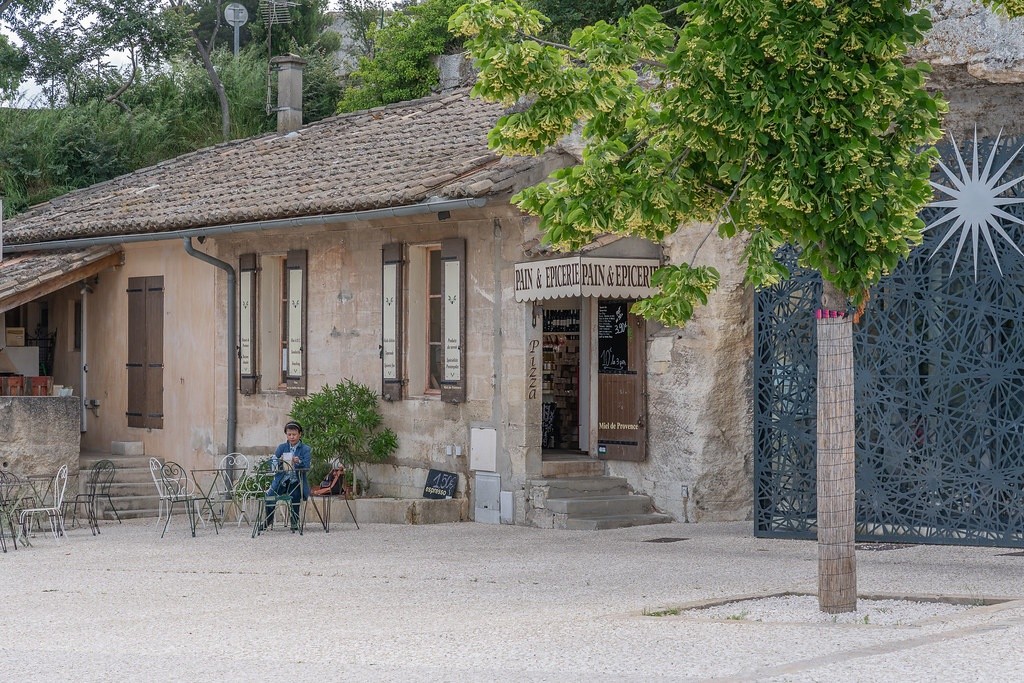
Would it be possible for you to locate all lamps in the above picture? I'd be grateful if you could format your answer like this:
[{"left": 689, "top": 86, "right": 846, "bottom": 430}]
[
  {"left": 438, "top": 211, "right": 451, "bottom": 221},
  {"left": 198, "top": 236, "right": 207, "bottom": 244}
]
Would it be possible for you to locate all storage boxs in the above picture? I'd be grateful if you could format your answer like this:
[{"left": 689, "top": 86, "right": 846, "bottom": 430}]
[{"left": 5, "top": 327, "right": 25, "bottom": 346}]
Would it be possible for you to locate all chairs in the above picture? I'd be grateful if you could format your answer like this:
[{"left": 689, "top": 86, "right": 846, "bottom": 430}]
[
  {"left": 15, "top": 464, "right": 70, "bottom": 545},
  {"left": 302, "top": 455, "right": 359, "bottom": 532},
  {"left": 206, "top": 452, "right": 250, "bottom": 528},
  {"left": 1, "top": 469, "right": 41, "bottom": 553},
  {"left": 149, "top": 457, "right": 206, "bottom": 533},
  {"left": 71, "top": 459, "right": 122, "bottom": 528},
  {"left": 252, "top": 459, "right": 304, "bottom": 538},
  {"left": 161, "top": 461, "right": 219, "bottom": 539},
  {"left": 59, "top": 462, "right": 102, "bottom": 536}
]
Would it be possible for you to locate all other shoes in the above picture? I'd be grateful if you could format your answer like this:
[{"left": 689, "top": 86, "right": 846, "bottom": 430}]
[
  {"left": 258, "top": 519, "right": 273, "bottom": 531},
  {"left": 290, "top": 517, "right": 298, "bottom": 530}
]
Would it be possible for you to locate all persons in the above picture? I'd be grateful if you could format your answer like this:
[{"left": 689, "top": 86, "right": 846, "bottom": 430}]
[{"left": 260, "top": 421, "right": 311, "bottom": 531}]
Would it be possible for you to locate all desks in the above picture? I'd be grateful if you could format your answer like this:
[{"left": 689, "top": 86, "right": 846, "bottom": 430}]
[
  {"left": 250, "top": 467, "right": 311, "bottom": 536},
  {"left": 190, "top": 468, "right": 250, "bottom": 535},
  {"left": 26, "top": 473, "right": 63, "bottom": 539}
]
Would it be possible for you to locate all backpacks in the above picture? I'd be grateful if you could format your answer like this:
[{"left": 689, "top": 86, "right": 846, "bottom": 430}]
[{"left": 314, "top": 467, "right": 345, "bottom": 495}]
